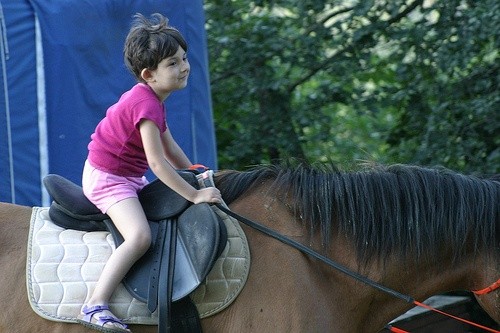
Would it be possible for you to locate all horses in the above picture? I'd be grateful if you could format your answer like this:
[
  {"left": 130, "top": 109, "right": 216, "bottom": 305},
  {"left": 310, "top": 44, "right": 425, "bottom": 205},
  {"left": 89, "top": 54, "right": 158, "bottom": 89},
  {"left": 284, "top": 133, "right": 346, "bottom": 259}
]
[{"left": 0, "top": 153, "right": 500, "bottom": 333}]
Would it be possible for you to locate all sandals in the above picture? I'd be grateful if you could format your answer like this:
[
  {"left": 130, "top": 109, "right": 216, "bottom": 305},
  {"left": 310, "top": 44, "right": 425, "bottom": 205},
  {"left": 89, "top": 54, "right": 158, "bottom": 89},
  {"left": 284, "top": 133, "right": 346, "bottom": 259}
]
[{"left": 76, "top": 304, "right": 132, "bottom": 333}]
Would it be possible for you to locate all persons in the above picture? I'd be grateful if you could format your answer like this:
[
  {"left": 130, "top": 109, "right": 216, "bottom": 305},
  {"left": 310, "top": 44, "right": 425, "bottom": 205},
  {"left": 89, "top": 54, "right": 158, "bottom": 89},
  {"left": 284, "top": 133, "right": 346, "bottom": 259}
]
[{"left": 76, "top": 12, "right": 222, "bottom": 333}]
